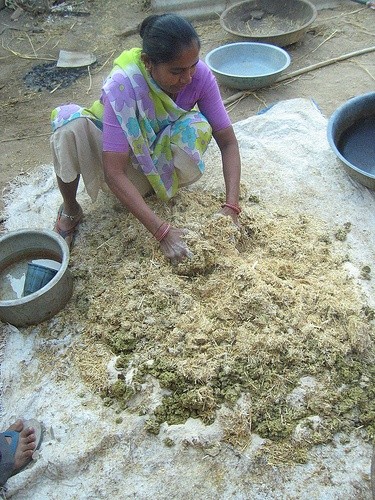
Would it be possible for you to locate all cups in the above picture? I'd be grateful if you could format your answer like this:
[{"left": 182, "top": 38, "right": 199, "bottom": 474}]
[{"left": 24, "top": 262, "right": 58, "bottom": 297}]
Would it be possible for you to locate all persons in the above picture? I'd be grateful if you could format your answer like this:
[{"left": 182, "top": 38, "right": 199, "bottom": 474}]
[
  {"left": 0, "top": 418, "right": 42, "bottom": 488},
  {"left": 49, "top": 15, "right": 241, "bottom": 264}
]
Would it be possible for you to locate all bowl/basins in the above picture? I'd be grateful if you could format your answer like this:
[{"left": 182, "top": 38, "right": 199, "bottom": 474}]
[
  {"left": 219, "top": 0, "right": 318, "bottom": 48},
  {"left": 0, "top": 227, "right": 73, "bottom": 327},
  {"left": 204, "top": 42, "right": 291, "bottom": 91},
  {"left": 327, "top": 91, "right": 375, "bottom": 191}
]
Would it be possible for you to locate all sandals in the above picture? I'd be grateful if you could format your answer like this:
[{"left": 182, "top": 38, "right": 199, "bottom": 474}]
[{"left": 52, "top": 201, "right": 83, "bottom": 251}]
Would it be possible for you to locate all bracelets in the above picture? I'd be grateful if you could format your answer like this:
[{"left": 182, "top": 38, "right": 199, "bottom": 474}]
[
  {"left": 221, "top": 204, "right": 241, "bottom": 214},
  {"left": 157, "top": 225, "right": 170, "bottom": 241},
  {"left": 153, "top": 222, "right": 165, "bottom": 236}
]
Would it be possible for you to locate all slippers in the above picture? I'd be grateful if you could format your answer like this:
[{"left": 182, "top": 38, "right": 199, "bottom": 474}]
[{"left": 0, "top": 418, "right": 44, "bottom": 492}]
